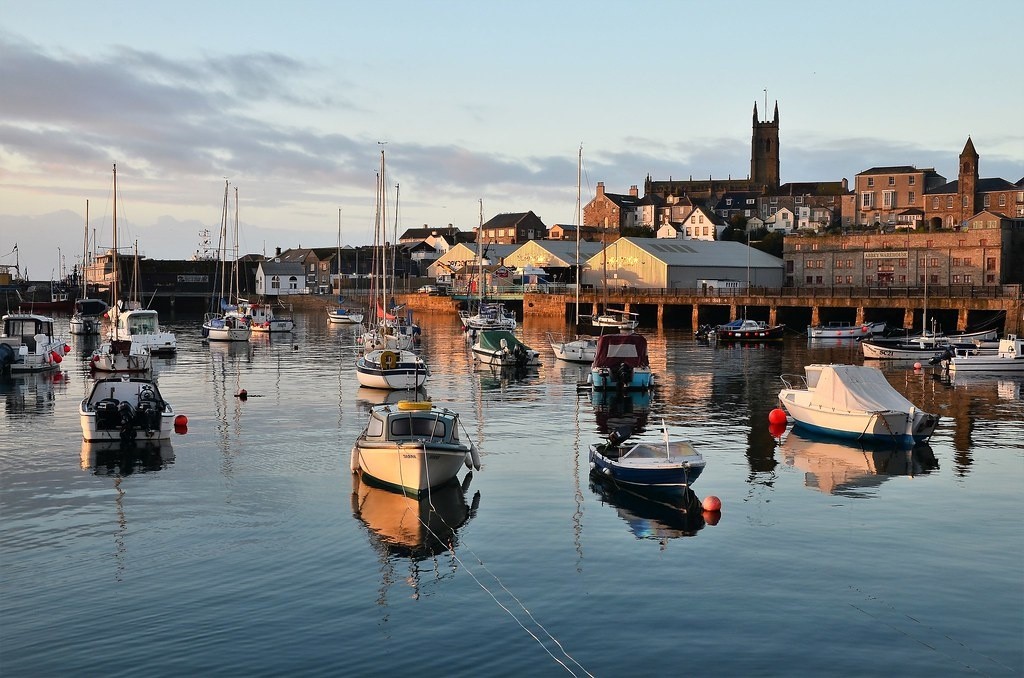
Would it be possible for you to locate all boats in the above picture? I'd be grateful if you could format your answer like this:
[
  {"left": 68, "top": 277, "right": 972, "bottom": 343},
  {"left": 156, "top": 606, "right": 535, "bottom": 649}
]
[
  {"left": 854, "top": 254, "right": 1024, "bottom": 361},
  {"left": 472, "top": 326, "right": 541, "bottom": 367},
  {"left": 776, "top": 364, "right": 941, "bottom": 446},
  {"left": 693, "top": 305, "right": 787, "bottom": 342},
  {"left": 585, "top": 332, "right": 656, "bottom": 390},
  {"left": 78, "top": 374, "right": 177, "bottom": 441},
  {"left": 589, "top": 418, "right": 706, "bottom": 497},
  {"left": 543, "top": 329, "right": 597, "bottom": 361},
  {"left": 807, "top": 321, "right": 888, "bottom": 338},
  {"left": 929, "top": 333, "right": 1024, "bottom": 372},
  {"left": 349, "top": 357, "right": 482, "bottom": 497}
]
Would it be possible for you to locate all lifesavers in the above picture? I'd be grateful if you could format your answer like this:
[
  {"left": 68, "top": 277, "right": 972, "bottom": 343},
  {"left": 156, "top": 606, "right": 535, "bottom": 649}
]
[
  {"left": 397, "top": 399, "right": 433, "bottom": 411},
  {"left": 380, "top": 327, "right": 392, "bottom": 338},
  {"left": 380, "top": 351, "right": 396, "bottom": 369}
]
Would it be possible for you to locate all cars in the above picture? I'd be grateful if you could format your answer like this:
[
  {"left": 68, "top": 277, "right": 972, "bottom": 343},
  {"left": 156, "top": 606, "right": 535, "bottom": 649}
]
[{"left": 417, "top": 285, "right": 438, "bottom": 294}]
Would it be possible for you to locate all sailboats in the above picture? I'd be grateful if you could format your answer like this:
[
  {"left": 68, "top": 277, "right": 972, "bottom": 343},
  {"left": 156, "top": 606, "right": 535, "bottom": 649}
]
[
  {"left": 456, "top": 196, "right": 516, "bottom": 336},
  {"left": 0, "top": 161, "right": 177, "bottom": 382},
  {"left": 573, "top": 139, "right": 638, "bottom": 337},
  {"left": 201, "top": 176, "right": 297, "bottom": 340},
  {"left": 325, "top": 208, "right": 365, "bottom": 322},
  {"left": 353, "top": 141, "right": 431, "bottom": 390}
]
